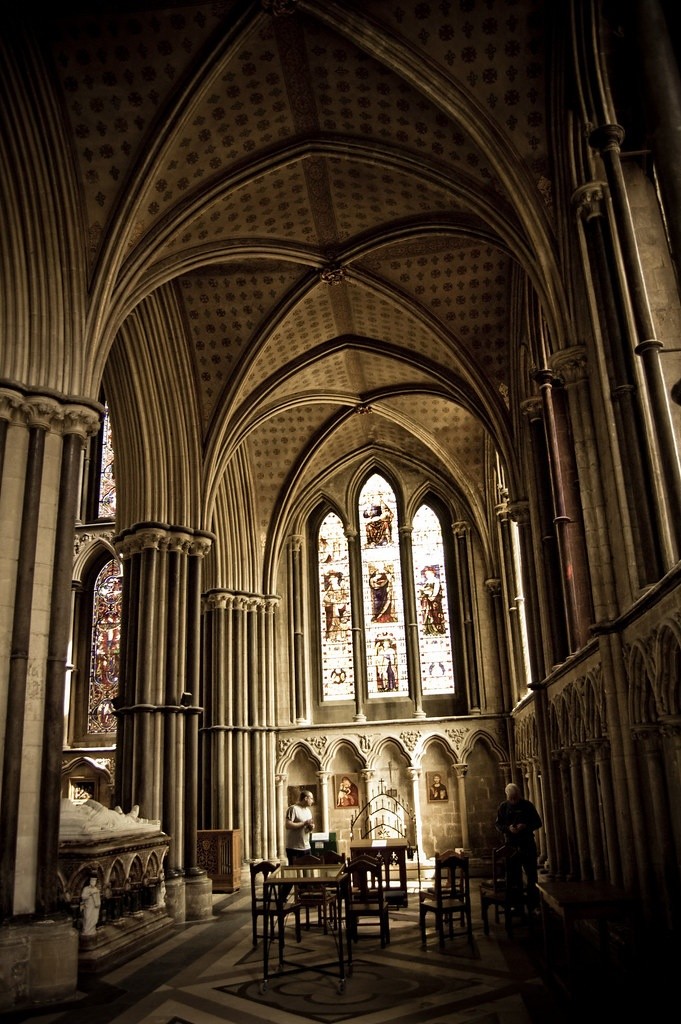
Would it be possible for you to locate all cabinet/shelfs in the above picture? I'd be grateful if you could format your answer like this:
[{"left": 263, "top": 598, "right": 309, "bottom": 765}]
[{"left": 197, "top": 830, "right": 242, "bottom": 892}]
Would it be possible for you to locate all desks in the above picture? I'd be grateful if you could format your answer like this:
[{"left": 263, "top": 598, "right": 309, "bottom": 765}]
[
  {"left": 264, "top": 864, "right": 352, "bottom": 994},
  {"left": 535, "top": 881, "right": 636, "bottom": 958}
]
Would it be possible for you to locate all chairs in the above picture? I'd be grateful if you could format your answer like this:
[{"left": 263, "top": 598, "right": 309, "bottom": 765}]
[
  {"left": 479, "top": 847, "right": 538, "bottom": 936},
  {"left": 250, "top": 846, "right": 407, "bottom": 949},
  {"left": 420, "top": 849, "right": 469, "bottom": 929},
  {"left": 420, "top": 857, "right": 472, "bottom": 951}
]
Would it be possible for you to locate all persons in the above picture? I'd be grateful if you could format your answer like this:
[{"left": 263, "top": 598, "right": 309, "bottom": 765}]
[
  {"left": 337, "top": 777, "right": 358, "bottom": 806},
  {"left": 61, "top": 797, "right": 140, "bottom": 829},
  {"left": 80, "top": 877, "right": 101, "bottom": 935},
  {"left": 430, "top": 774, "right": 448, "bottom": 800},
  {"left": 496, "top": 782, "right": 543, "bottom": 916},
  {"left": 277, "top": 790, "right": 315, "bottom": 901}
]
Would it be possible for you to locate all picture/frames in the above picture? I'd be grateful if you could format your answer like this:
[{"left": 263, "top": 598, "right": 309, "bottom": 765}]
[
  {"left": 334, "top": 773, "right": 360, "bottom": 809},
  {"left": 288, "top": 784, "right": 317, "bottom": 807},
  {"left": 426, "top": 771, "right": 449, "bottom": 802}
]
